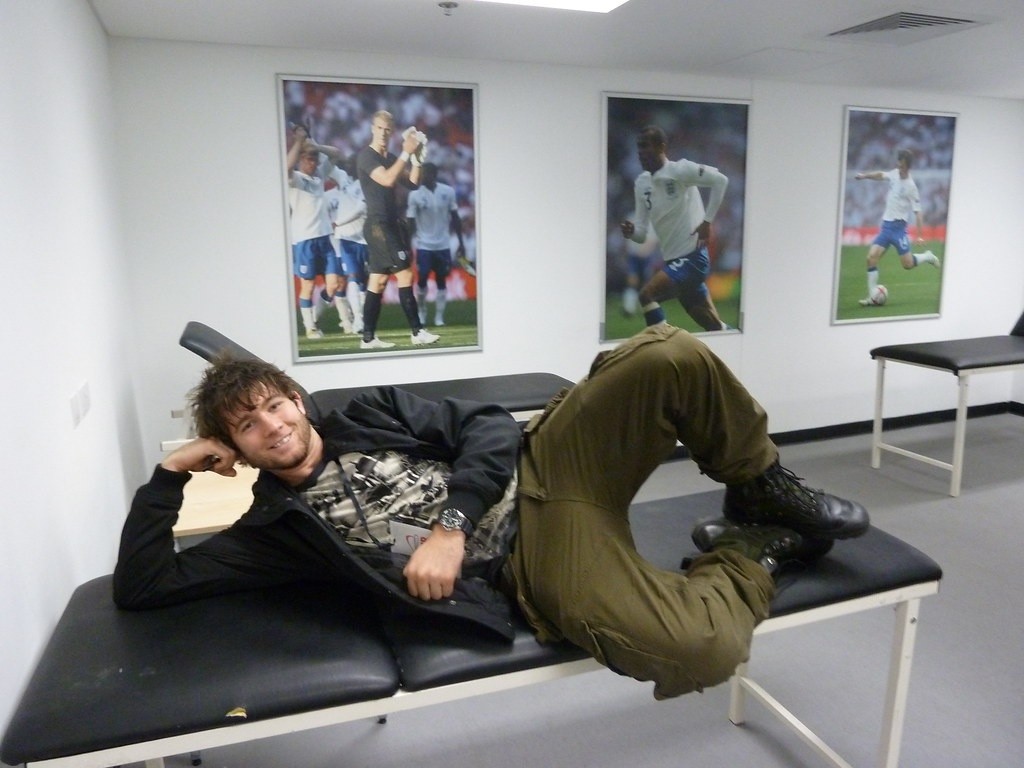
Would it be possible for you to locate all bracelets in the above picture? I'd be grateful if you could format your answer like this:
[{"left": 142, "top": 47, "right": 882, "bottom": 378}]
[{"left": 399, "top": 150, "right": 410, "bottom": 162}]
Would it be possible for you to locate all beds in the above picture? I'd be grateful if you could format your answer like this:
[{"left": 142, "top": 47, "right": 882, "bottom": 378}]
[
  {"left": 178, "top": 322, "right": 579, "bottom": 424},
  {"left": 0, "top": 490, "right": 943, "bottom": 768},
  {"left": 868, "top": 313, "right": 1024, "bottom": 496}
]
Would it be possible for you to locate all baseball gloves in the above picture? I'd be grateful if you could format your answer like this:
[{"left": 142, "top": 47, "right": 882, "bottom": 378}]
[{"left": 401, "top": 125, "right": 428, "bottom": 168}]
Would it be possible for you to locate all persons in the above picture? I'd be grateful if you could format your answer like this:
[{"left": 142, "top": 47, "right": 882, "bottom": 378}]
[
  {"left": 855, "top": 149, "right": 940, "bottom": 306},
  {"left": 619, "top": 124, "right": 733, "bottom": 333},
  {"left": 621, "top": 211, "right": 659, "bottom": 318},
  {"left": 357, "top": 110, "right": 440, "bottom": 349},
  {"left": 111, "top": 324, "right": 870, "bottom": 705},
  {"left": 285, "top": 124, "right": 369, "bottom": 339},
  {"left": 407, "top": 164, "right": 466, "bottom": 327}
]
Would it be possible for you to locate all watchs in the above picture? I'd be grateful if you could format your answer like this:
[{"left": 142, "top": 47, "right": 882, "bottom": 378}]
[{"left": 440, "top": 507, "right": 473, "bottom": 538}]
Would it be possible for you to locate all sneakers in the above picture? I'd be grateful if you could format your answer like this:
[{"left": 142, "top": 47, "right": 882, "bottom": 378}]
[
  {"left": 411, "top": 329, "right": 441, "bottom": 345},
  {"left": 692, "top": 514, "right": 834, "bottom": 576},
  {"left": 360, "top": 334, "right": 396, "bottom": 349},
  {"left": 723, "top": 456, "right": 868, "bottom": 539}
]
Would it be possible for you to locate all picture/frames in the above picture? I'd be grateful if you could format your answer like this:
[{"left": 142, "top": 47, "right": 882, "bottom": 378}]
[
  {"left": 829, "top": 106, "right": 957, "bottom": 326},
  {"left": 598, "top": 91, "right": 754, "bottom": 346},
  {"left": 276, "top": 73, "right": 482, "bottom": 363}
]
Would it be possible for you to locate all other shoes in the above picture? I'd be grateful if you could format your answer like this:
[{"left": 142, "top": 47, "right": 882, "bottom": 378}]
[
  {"left": 418, "top": 311, "right": 426, "bottom": 325},
  {"left": 926, "top": 250, "right": 939, "bottom": 268},
  {"left": 435, "top": 314, "right": 444, "bottom": 326},
  {"left": 338, "top": 317, "right": 353, "bottom": 334},
  {"left": 354, "top": 315, "right": 364, "bottom": 332},
  {"left": 859, "top": 297, "right": 876, "bottom": 306},
  {"left": 306, "top": 328, "right": 323, "bottom": 339}
]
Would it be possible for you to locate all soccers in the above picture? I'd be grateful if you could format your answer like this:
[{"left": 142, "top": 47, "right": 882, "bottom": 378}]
[{"left": 871, "top": 285, "right": 888, "bottom": 305}]
